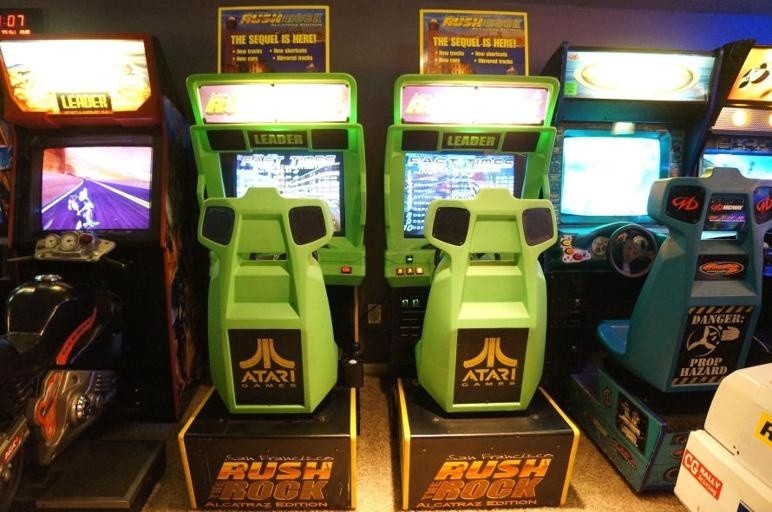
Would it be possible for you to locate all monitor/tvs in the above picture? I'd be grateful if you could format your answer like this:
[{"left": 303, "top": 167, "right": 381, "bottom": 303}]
[
  {"left": 699, "top": 148, "right": 772, "bottom": 181},
  {"left": 401, "top": 153, "right": 517, "bottom": 240},
  {"left": 214, "top": 149, "right": 343, "bottom": 240},
  {"left": 35, "top": 137, "right": 153, "bottom": 236},
  {"left": 562, "top": 137, "right": 664, "bottom": 219}
]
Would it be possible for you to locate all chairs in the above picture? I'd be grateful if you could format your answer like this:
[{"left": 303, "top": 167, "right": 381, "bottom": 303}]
[
  {"left": 195, "top": 183, "right": 343, "bottom": 418},
  {"left": 591, "top": 166, "right": 772, "bottom": 396},
  {"left": 408, "top": 184, "right": 560, "bottom": 416}
]
